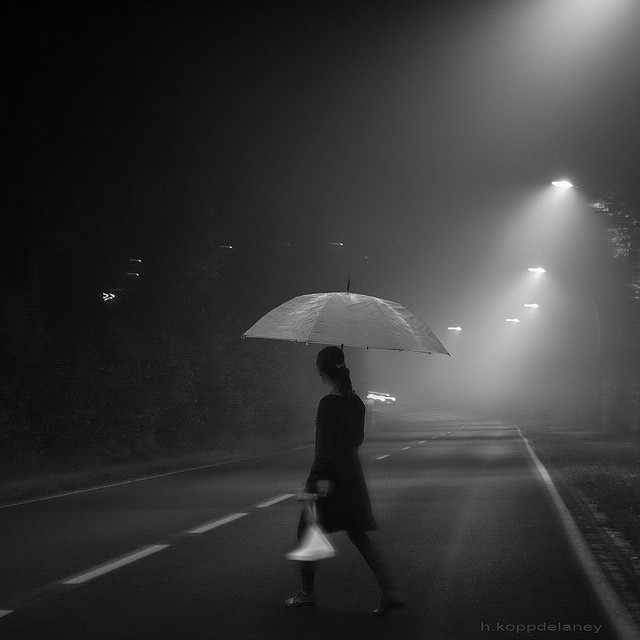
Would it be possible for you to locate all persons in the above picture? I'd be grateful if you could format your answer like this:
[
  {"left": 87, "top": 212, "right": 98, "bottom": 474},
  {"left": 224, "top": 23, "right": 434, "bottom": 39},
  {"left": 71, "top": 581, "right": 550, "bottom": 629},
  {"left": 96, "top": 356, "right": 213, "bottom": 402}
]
[{"left": 285, "top": 347, "right": 406, "bottom": 616}]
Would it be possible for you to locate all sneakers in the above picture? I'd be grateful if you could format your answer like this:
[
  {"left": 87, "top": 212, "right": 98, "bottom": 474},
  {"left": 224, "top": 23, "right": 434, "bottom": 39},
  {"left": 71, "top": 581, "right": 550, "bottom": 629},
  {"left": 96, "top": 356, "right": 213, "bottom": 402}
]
[
  {"left": 285, "top": 590, "right": 317, "bottom": 608},
  {"left": 370, "top": 592, "right": 404, "bottom": 615}
]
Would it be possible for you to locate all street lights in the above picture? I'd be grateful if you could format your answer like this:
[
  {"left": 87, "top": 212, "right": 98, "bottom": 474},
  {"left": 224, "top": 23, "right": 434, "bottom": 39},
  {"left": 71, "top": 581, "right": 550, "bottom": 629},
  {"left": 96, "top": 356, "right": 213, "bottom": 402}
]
[{"left": 527, "top": 262, "right": 603, "bottom": 439}]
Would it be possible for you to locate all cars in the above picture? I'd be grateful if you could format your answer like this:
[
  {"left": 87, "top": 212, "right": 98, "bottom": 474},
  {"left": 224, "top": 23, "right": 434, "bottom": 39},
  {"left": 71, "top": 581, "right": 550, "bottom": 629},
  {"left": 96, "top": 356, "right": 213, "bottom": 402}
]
[{"left": 367, "top": 391, "right": 397, "bottom": 406}]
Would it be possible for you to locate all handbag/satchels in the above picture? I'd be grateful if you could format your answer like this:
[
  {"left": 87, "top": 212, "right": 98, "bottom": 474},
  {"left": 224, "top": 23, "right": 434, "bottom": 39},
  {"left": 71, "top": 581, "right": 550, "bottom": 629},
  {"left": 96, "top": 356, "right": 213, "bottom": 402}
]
[{"left": 297, "top": 479, "right": 329, "bottom": 499}]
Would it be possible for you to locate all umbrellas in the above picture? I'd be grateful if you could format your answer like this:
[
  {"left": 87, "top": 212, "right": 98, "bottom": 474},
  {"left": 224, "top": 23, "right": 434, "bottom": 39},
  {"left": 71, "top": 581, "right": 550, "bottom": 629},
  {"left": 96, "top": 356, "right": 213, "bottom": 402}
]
[{"left": 239, "top": 278, "right": 451, "bottom": 357}]
